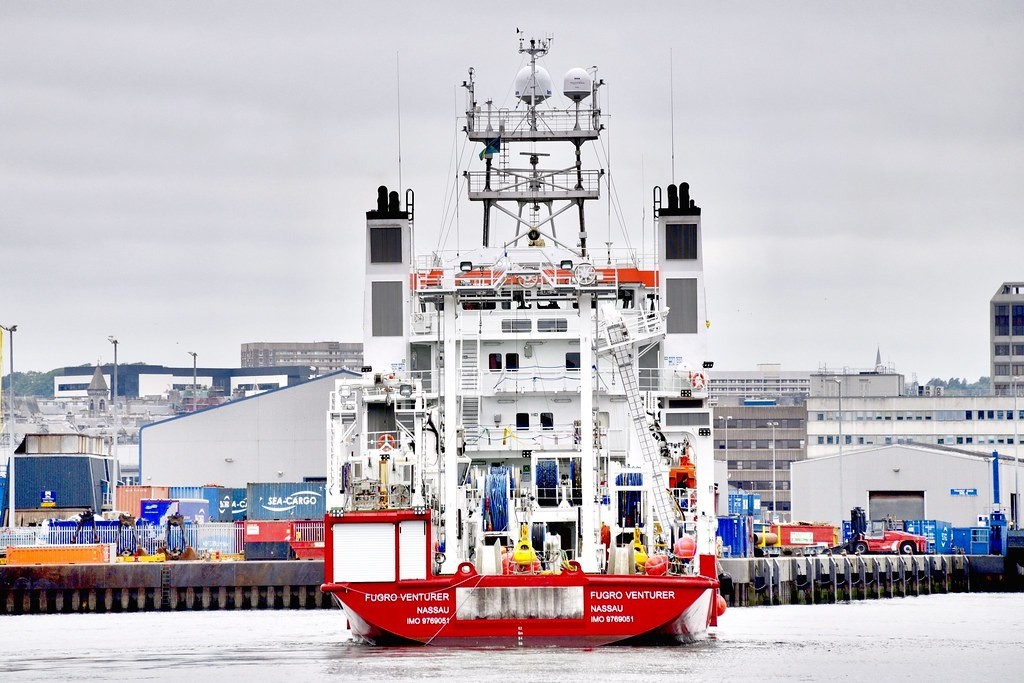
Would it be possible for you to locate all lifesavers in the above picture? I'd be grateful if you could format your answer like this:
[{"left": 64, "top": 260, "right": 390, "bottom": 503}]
[
  {"left": 377, "top": 434, "right": 395, "bottom": 452},
  {"left": 693, "top": 373, "right": 705, "bottom": 390}
]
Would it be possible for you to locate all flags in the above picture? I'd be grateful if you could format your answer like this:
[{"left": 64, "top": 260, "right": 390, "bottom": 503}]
[{"left": 479, "top": 136, "right": 500, "bottom": 161}]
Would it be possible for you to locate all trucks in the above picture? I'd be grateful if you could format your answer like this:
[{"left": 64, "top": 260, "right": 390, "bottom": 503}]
[{"left": 753, "top": 522, "right": 838, "bottom": 557}]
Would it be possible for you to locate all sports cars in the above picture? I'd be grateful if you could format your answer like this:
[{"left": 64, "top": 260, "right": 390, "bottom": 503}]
[{"left": 853, "top": 530, "right": 927, "bottom": 555}]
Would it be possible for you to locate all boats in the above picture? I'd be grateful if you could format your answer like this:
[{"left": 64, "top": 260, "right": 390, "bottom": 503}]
[{"left": 320, "top": 27, "right": 735, "bottom": 649}]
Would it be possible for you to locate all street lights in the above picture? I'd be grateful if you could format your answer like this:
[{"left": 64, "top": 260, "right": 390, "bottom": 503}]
[
  {"left": 108, "top": 335, "right": 119, "bottom": 509},
  {"left": 767, "top": 420, "right": 778, "bottom": 523},
  {"left": 719, "top": 415, "right": 734, "bottom": 461},
  {"left": 187, "top": 351, "right": 198, "bottom": 411},
  {"left": 0, "top": 324, "right": 18, "bottom": 532},
  {"left": 835, "top": 379, "right": 842, "bottom": 454}
]
